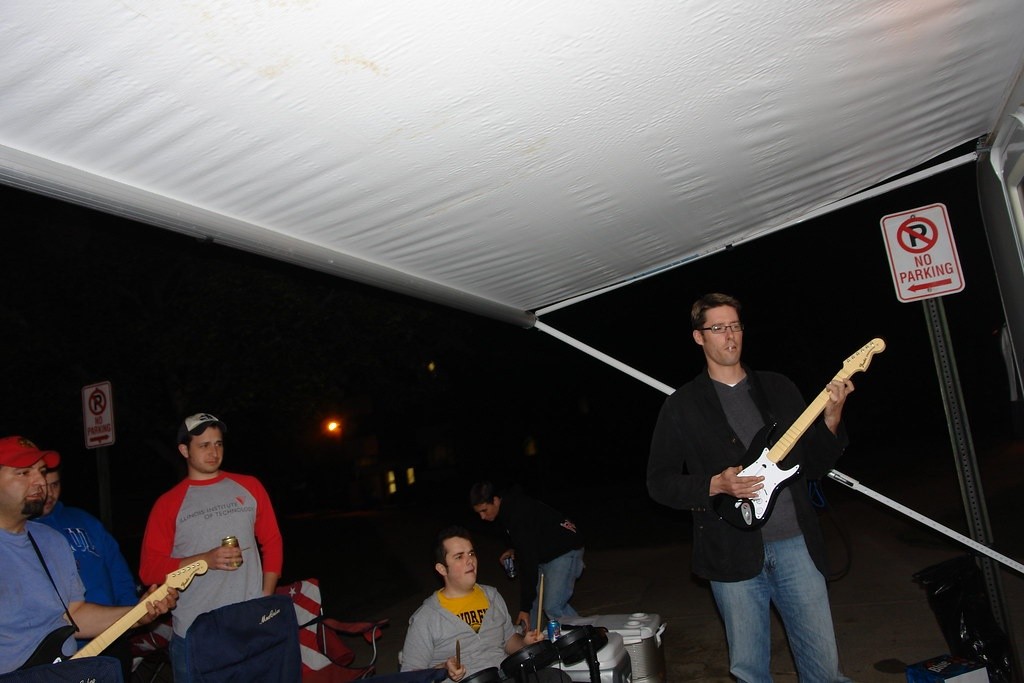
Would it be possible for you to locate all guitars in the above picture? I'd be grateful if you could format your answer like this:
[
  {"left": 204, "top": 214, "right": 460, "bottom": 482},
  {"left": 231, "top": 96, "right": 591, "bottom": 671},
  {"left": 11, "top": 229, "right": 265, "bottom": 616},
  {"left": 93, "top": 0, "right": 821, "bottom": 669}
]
[
  {"left": 712, "top": 338, "right": 886, "bottom": 531},
  {"left": 0, "top": 557, "right": 209, "bottom": 677}
]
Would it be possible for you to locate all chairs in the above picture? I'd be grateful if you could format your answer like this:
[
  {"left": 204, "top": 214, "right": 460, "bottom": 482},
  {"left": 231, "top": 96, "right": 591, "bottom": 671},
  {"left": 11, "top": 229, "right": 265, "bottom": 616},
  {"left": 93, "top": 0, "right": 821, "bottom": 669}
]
[
  {"left": 274, "top": 578, "right": 390, "bottom": 683},
  {"left": 185, "top": 593, "right": 303, "bottom": 683},
  {"left": 0, "top": 657, "right": 124, "bottom": 683}
]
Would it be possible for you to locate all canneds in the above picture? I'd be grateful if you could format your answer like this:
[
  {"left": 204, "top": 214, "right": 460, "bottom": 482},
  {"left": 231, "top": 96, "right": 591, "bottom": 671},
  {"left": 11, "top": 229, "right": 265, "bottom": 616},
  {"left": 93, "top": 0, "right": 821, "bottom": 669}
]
[
  {"left": 222, "top": 536, "right": 243, "bottom": 568},
  {"left": 504, "top": 556, "right": 516, "bottom": 577},
  {"left": 547, "top": 620, "right": 561, "bottom": 643}
]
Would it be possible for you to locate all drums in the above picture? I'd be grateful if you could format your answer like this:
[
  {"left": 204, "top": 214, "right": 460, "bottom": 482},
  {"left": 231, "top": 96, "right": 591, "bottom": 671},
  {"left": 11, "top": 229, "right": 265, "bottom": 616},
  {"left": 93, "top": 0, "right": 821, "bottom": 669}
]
[
  {"left": 555, "top": 625, "right": 609, "bottom": 666},
  {"left": 459, "top": 667, "right": 500, "bottom": 682},
  {"left": 500, "top": 640, "right": 560, "bottom": 680}
]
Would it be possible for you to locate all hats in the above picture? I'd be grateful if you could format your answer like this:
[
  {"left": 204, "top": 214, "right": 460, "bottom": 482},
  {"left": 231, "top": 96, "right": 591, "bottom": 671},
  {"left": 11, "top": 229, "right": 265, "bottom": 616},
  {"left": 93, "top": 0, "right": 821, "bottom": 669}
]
[
  {"left": 0, "top": 434, "right": 60, "bottom": 468},
  {"left": 177, "top": 413, "right": 227, "bottom": 447}
]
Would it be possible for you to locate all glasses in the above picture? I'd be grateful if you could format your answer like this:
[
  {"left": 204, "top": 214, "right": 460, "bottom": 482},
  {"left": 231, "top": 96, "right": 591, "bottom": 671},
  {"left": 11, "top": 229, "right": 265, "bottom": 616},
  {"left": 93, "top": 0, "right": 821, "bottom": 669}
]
[{"left": 699, "top": 324, "right": 744, "bottom": 334}]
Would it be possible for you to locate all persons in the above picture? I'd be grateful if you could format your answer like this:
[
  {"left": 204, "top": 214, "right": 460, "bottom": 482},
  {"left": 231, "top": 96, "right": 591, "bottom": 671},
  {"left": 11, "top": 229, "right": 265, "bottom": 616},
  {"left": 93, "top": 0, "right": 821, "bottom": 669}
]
[
  {"left": 645, "top": 293, "right": 856, "bottom": 683},
  {"left": 0, "top": 437, "right": 180, "bottom": 683},
  {"left": 398, "top": 524, "right": 572, "bottom": 683},
  {"left": 139, "top": 412, "right": 284, "bottom": 683},
  {"left": 466, "top": 477, "right": 585, "bottom": 633}
]
